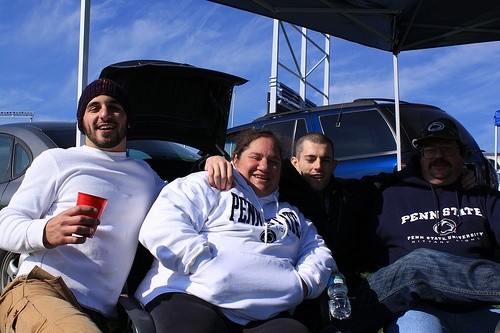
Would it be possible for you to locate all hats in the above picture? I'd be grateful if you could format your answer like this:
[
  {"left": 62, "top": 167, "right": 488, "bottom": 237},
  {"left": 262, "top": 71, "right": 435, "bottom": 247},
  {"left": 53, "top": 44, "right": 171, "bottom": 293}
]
[
  {"left": 77, "top": 78, "right": 133, "bottom": 135},
  {"left": 415, "top": 118, "right": 461, "bottom": 144}
]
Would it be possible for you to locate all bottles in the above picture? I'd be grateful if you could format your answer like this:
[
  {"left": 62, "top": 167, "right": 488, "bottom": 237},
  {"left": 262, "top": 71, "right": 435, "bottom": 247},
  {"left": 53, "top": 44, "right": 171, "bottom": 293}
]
[{"left": 328, "top": 270, "right": 351, "bottom": 320}]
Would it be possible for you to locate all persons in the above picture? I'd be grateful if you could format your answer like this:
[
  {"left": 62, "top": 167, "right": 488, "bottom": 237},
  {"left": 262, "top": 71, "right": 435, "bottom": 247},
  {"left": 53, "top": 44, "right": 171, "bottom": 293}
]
[
  {"left": 0, "top": 79, "right": 172, "bottom": 333},
  {"left": 190, "top": 132, "right": 500, "bottom": 333},
  {"left": 133, "top": 128, "right": 335, "bottom": 333},
  {"left": 372, "top": 117, "right": 500, "bottom": 333}
]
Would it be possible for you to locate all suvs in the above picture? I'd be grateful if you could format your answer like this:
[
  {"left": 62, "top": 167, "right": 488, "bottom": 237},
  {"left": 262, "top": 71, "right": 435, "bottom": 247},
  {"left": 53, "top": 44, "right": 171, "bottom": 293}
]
[{"left": 218, "top": 95, "right": 499, "bottom": 190}]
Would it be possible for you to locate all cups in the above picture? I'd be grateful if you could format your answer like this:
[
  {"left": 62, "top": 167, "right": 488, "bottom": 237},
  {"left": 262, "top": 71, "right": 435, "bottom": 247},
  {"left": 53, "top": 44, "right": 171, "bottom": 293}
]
[{"left": 72, "top": 192, "right": 108, "bottom": 238}]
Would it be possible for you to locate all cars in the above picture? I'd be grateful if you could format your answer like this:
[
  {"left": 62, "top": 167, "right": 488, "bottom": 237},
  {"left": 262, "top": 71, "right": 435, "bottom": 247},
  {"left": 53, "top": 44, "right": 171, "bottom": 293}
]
[{"left": 0, "top": 59, "right": 250, "bottom": 289}]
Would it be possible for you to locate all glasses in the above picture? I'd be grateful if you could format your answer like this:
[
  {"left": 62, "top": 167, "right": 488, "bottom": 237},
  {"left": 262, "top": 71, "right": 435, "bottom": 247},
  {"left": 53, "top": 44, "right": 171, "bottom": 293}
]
[{"left": 421, "top": 145, "right": 457, "bottom": 158}]
